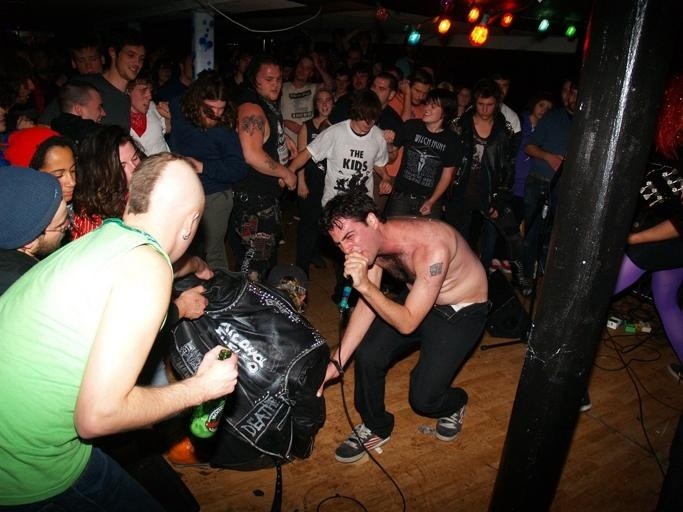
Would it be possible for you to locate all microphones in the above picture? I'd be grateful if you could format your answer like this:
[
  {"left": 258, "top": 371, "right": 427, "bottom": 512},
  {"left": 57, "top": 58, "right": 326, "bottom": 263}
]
[{"left": 338, "top": 275, "right": 353, "bottom": 310}]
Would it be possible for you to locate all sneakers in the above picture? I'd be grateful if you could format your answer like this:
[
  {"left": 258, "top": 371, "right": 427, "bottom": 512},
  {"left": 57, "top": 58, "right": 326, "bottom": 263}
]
[
  {"left": 336, "top": 423, "right": 391, "bottom": 463},
  {"left": 436, "top": 406, "right": 465, "bottom": 440}
]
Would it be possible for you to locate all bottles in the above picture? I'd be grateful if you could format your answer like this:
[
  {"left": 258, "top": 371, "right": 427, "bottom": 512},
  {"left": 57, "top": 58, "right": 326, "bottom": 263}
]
[{"left": 188, "top": 346, "right": 232, "bottom": 438}]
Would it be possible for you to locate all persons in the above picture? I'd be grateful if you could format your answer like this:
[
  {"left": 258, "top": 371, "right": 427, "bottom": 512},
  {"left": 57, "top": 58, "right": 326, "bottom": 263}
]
[
  {"left": 0, "top": 150, "right": 243, "bottom": 509},
  {"left": 0, "top": 30, "right": 608, "bottom": 466},
  {"left": 613, "top": 86, "right": 682, "bottom": 380}
]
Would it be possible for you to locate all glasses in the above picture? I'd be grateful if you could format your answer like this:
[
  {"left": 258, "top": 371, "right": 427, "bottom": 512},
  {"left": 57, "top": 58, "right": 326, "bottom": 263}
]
[{"left": 43, "top": 205, "right": 79, "bottom": 232}]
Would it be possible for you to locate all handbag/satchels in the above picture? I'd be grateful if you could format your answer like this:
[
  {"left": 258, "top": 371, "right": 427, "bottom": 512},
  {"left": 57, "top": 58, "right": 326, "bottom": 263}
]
[{"left": 170, "top": 271, "right": 327, "bottom": 470}]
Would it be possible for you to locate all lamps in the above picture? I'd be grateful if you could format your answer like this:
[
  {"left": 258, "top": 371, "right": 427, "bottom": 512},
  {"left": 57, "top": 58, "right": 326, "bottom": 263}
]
[{"left": 374, "top": 0, "right": 579, "bottom": 47}]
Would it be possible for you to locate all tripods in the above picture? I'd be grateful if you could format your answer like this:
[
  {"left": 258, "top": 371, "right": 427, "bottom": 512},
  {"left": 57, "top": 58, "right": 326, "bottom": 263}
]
[{"left": 487, "top": 201, "right": 571, "bottom": 351}]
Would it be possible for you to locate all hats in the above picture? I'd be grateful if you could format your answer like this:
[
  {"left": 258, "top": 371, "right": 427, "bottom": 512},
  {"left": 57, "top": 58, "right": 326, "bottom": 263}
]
[
  {"left": 7, "top": 129, "right": 61, "bottom": 166},
  {"left": 0, "top": 164, "right": 62, "bottom": 250}
]
